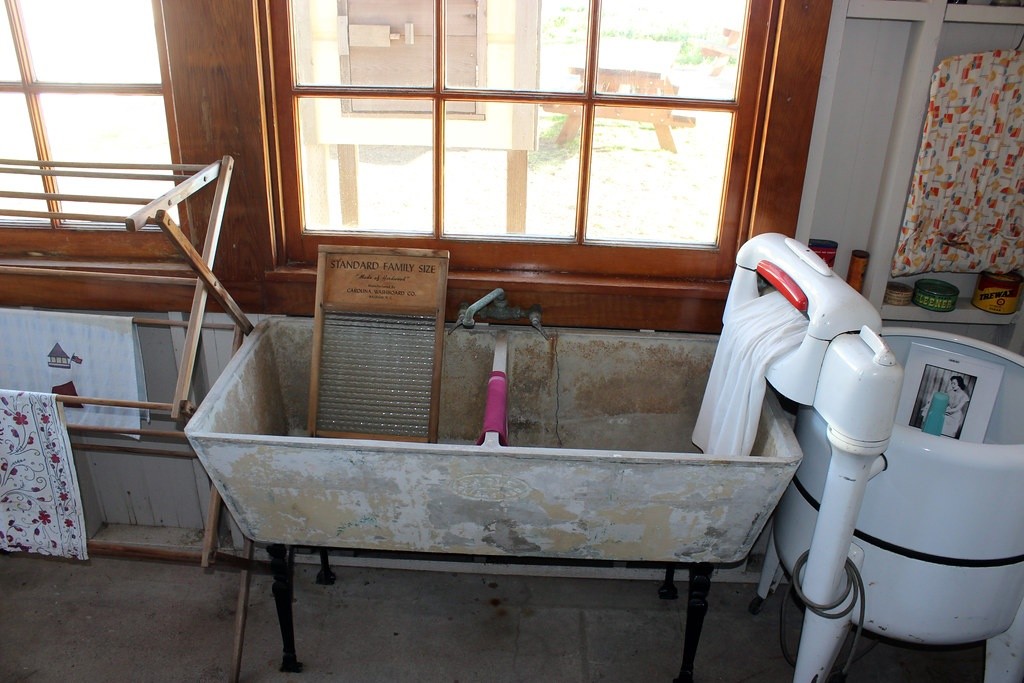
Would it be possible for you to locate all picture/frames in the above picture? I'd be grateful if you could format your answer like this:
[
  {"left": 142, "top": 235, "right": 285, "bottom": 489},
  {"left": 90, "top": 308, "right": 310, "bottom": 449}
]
[{"left": 889, "top": 342, "right": 1006, "bottom": 446}]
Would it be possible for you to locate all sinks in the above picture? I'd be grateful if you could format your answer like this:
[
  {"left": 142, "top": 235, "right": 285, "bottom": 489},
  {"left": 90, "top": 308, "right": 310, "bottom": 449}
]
[{"left": 180, "top": 314, "right": 807, "bottom": 566}]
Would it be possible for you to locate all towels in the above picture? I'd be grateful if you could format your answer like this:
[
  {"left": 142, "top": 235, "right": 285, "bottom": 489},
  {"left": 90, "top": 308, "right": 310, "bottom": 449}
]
[
  {"left": 0, "top": 389, "right": 90, "bottom": 562},
  {"left": 690, "top": 289, "right": 809, "bottom": 456},
  {"left": 1, "top": 308, "right": 151, "bottom": 441}
]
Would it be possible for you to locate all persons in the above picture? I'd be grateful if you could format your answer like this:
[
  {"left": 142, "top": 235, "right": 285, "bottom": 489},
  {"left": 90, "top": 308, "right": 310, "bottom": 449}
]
[{"left": 927, "top": 375, "right": 971, "bottom": 438}]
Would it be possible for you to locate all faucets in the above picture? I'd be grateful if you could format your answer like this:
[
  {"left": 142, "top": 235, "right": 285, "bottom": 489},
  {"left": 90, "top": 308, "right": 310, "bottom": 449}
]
[{"left": 462, "top": 287, "right": 508, "bottom": 329}]
